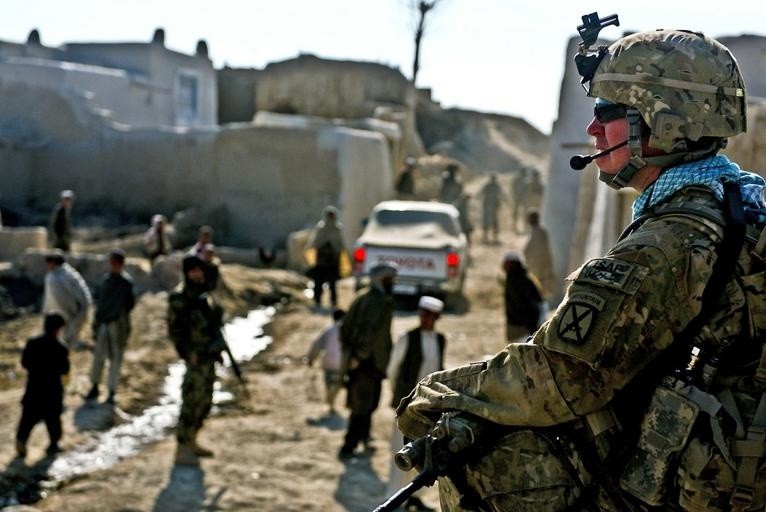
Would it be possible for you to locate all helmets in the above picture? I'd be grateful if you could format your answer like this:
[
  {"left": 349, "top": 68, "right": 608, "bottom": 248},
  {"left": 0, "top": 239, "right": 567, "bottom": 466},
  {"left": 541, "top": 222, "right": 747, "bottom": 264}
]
[{"left": 587, "top": 30, "right": 747, "bottom": 153}]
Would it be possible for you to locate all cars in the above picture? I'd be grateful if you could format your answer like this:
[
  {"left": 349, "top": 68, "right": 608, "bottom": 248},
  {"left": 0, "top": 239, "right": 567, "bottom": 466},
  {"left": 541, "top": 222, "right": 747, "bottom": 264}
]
[{"left": 353, "top": 199, "right": 472, "bottom": 310}]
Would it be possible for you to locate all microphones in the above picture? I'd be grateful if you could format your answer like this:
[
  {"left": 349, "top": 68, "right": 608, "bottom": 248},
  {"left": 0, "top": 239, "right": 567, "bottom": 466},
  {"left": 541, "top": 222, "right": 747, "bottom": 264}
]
[{"left": 569, "top": 131, "right": 645, "bottom": 170}]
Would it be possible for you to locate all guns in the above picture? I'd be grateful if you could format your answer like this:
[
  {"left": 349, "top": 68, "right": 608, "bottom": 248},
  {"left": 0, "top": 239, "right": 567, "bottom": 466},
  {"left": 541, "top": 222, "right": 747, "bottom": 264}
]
[
  {"left": 200, "top": 292, "right": 242, "bottom": 378},
  {"left": 371, "top": 412, "right": 502, "bottom": 512}
]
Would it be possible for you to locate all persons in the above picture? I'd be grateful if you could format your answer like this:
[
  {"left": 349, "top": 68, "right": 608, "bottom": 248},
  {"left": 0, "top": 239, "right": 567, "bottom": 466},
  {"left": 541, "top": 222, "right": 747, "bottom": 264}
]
[
  {"left": 304, "top": 204, "right": 346, "bottom": 312},
  {"left": 503, "top": 253, "right": 543, "bottom": 347},
  {"left": 395, "top": 158, "right": 545, "bottom": 245},
  {"left": 16, "top": 187, "right": 221, "bottom": 468},
  {"left": 389, "top": 293, "right": 447, "bottom": 448},
  {"left": 524, "top": 211, "right": 555, "bottom": 291},
  {"left": 335, "top": 261, "right": 399, "bottom": 459},
  {"left": 307, "top": 309, "right": 345, "bottom": 414},
  {"left": 393, "top": 28, "right": 766, "bottom": 511}
]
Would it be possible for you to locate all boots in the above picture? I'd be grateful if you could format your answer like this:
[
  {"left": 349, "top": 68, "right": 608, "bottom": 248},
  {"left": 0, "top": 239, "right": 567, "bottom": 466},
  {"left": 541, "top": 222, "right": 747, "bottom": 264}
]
[{"left": 176, "top": 441, "right": 213, "bottom": 467}]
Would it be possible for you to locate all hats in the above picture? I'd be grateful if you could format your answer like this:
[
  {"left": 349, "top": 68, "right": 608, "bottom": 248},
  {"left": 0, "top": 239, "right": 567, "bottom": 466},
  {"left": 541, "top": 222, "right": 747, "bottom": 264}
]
[{"left": 419, "top": 296, "right": 444, "bottom": 313}]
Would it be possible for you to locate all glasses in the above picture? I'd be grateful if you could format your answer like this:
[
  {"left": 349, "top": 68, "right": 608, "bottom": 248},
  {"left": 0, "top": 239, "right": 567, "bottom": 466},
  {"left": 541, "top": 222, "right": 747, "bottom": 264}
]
[{"left": 594, "top": 97, "right": 627, "bottom": 123}]
